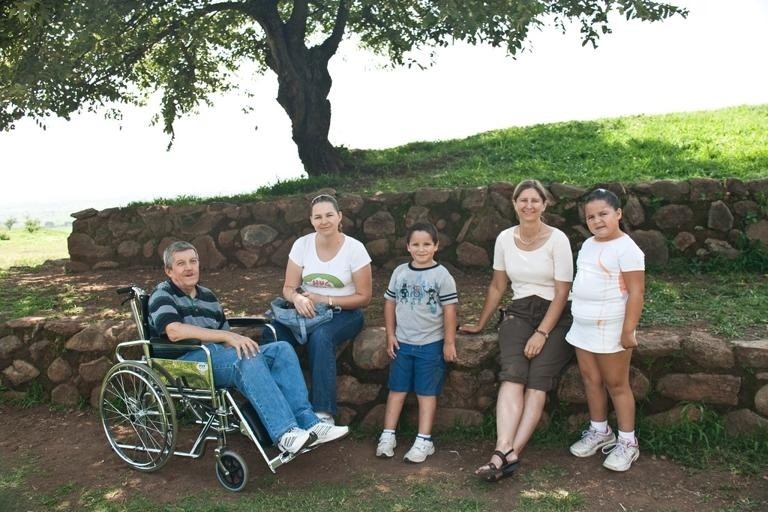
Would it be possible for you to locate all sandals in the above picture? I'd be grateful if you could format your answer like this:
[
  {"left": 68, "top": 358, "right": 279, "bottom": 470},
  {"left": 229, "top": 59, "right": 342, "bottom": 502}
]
[{"left": 472, "top": 449, "right": 518, "bottom": 482}]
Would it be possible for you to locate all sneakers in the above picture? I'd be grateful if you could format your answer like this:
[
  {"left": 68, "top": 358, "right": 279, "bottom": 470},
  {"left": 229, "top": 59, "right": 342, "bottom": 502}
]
[
  {"left": 403, "top": 437, "right": 435, "bottom": 464},
  {"left": 314, "top": 413, "right": 334, "bottom": 426},
  {"left": 304, "top": 423, "right": 350, "bottom": 449},
  {"left": 277, "top": 427, "right": 317, "bottom": 455},
  {"left": 569, "top": 425, "right": 616, "bottom": 458},
  {"left": 602, "top": 436, "right": 639, "bottom": 472},
  {"left": 375, "top": 432, "right": 395, "bottom": 458}
]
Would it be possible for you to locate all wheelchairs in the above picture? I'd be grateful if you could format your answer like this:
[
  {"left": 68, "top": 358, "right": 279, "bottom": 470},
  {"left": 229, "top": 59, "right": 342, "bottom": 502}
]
[{"left": 97, "top": 282, "right": 336, "bottom": 494}]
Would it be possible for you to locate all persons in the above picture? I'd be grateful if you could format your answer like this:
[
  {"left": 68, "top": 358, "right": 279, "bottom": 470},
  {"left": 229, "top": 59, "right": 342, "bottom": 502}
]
[
  {"left": 563, "top": 188, "right": 646, "bottom": 473},
  {"left": 148, "top": 239, "right": 353, "bottom": 456},
  {"left": 258, "top": 193, "right": 374, "bottom": 428},
  {"left": 374, "top": 222, "right": 459, "bottom": 465},
  {"left": 458, "top": 179, "right": 576, "bottom": 483}
]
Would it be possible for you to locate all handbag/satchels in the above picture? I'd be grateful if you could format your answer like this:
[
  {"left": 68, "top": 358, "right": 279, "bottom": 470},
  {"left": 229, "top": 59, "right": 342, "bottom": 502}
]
[{"left": 270, "top": 298, "right": 333, "bottom": 335}]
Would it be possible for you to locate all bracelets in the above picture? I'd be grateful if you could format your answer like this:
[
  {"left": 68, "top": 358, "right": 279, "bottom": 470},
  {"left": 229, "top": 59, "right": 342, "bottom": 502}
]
[
  {"left": 328, "top": 295, "right": 334, "bottom": 307},
  {"left": 535, "top": 328, "right": 549, "bottom": 339}
]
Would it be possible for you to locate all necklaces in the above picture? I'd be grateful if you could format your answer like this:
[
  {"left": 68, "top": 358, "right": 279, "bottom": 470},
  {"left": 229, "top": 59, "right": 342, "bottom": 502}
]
[{"left": 516, "top": 222, "right": 543, "bottom": 246}]
